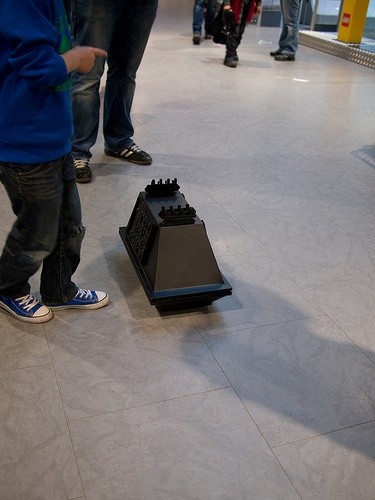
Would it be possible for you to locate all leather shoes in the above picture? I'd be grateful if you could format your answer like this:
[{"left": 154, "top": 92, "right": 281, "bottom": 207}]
[
  {"left": 274, "top": 54, "right": 295, "bottom": 61},
  {"left": 270, "top": 51, "right": 281, "bottom": 56}
]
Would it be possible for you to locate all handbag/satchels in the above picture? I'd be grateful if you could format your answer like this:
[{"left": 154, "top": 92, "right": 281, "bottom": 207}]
[{"left": 212, "top": 9, "right": 237, "bottom": 44}]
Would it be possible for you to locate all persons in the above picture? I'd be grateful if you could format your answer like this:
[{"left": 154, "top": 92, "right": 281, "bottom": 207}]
[
  {"left": 192, "top": 0, "right": 262, "bottom": 67},
  {"left": 270, "top": 0, "right": 301, "bottom": 61},
  {"left": 0, "top": 0, "right": 110, "bottom": 323},
  {"left": 71, "top": 0, "right": 158, "bottom": 182}
]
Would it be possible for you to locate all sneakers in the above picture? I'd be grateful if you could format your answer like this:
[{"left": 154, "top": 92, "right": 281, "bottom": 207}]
[
  {"left": 104, "top": 142, "right": 152, "bottom": 165},
  {"left": 41, "top": 288, "right": 110, "bottom": 312},
  {"left": 73, "top": 159, "right": 92, "bottom": 184},
  {"left": 193, "top": 31, "right": 200, "bottom": 44},
  {"left": 224, "top": 55, "right": 239, "bottom": 68},
  {"left": 0, "top": 294, "right": 53, "bottom": 324},
  {"left": 205, "top": 34, "right": 212, "bottom": 39}
]
